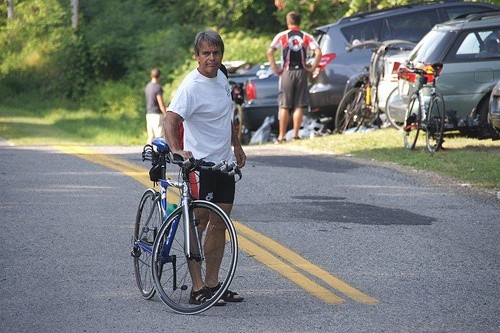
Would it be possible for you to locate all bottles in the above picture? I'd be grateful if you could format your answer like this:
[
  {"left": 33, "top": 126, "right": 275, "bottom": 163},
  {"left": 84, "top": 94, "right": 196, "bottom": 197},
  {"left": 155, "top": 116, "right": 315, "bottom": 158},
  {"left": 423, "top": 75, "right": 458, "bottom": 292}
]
[{"left": 161, "top": 203, "right": 177, "bottom": 222}]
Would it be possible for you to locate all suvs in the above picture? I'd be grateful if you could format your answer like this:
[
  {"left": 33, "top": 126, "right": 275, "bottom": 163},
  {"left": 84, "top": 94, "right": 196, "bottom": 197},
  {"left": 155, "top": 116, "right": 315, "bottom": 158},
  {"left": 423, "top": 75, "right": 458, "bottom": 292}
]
[
  {"left": 404, "top": 10, "right": 500, "bottom": 139},
  {"left": 313, "top": 2, "right": 500, "bottom": 110}
]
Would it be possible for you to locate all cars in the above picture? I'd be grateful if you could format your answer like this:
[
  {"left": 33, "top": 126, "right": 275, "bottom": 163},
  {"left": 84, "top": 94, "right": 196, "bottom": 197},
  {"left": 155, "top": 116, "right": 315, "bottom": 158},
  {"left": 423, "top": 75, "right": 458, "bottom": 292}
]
[
  {"left": 347, "top": 39, "right": 416, "bottom": 114},
  {"left": 228, "top": 60, "right": 306, "bottom": 144}
]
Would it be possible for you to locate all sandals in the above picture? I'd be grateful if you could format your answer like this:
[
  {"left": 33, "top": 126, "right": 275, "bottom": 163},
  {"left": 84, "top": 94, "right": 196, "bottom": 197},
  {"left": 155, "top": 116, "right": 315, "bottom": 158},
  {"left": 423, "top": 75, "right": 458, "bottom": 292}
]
[
  {"left": 189, "top": 282, "right": 242, "bottom": 305},
  {"left": 275, "top": 137, "right": 300, "bottom": 143}
]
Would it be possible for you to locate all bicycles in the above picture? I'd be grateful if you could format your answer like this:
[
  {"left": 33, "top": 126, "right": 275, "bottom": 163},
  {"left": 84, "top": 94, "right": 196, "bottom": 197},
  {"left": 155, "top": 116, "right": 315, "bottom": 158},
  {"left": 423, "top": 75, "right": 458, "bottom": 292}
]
[
  {"left": 336, "top": 79, "right": 378, "bottom": 128},
  {"left": 131, "top": 138, "right": 244, "bottom": 315},
  {"left": 401, "top": 63, "right": 446, "bottom": 152}
]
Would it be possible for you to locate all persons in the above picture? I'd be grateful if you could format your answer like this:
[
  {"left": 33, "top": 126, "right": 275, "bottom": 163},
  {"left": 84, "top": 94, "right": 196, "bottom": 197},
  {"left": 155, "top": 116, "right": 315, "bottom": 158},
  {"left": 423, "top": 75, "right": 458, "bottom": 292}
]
[
  {"left": 267, "top": 12, "right": 322, "bottom": 144},
  {"left": 162, "top": 30, "right": 247, "bottom": 306},
  {"left": 144, "top": 68, "right": 166, "bottom": 145}
]
